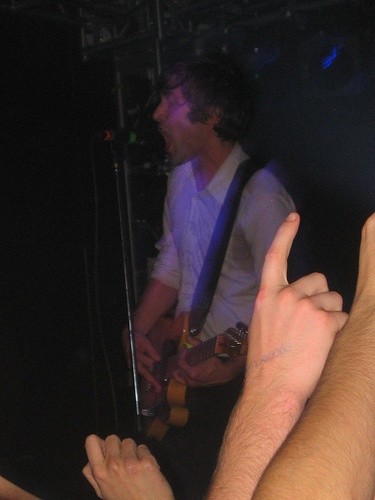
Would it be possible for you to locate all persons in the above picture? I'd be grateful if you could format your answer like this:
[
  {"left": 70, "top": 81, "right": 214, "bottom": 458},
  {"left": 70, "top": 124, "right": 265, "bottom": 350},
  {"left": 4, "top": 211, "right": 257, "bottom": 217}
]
[
  {"left": 0, "top": 210, "right": 344, "bottom": 500},
  {"left": 121, "top": 54, "right": 295, "bottom": 499},
  {"left": 250, "top": 212, "right": 374, "bottom": 499},
  {"left": 81, "top": 426, "right": 170, "bottom": 500}
]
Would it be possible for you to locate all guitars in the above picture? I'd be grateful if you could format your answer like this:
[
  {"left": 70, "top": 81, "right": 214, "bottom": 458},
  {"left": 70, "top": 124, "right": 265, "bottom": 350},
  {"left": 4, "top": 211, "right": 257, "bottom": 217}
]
[{"left": 140, "top": 312, "right": 248, "bottom": 441}]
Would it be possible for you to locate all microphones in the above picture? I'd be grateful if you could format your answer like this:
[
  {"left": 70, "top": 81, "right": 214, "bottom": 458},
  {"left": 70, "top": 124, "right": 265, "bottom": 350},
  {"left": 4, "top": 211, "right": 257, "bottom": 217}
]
[{"left": 104, "top": 127, "right": 164, "bottom": 147}]
